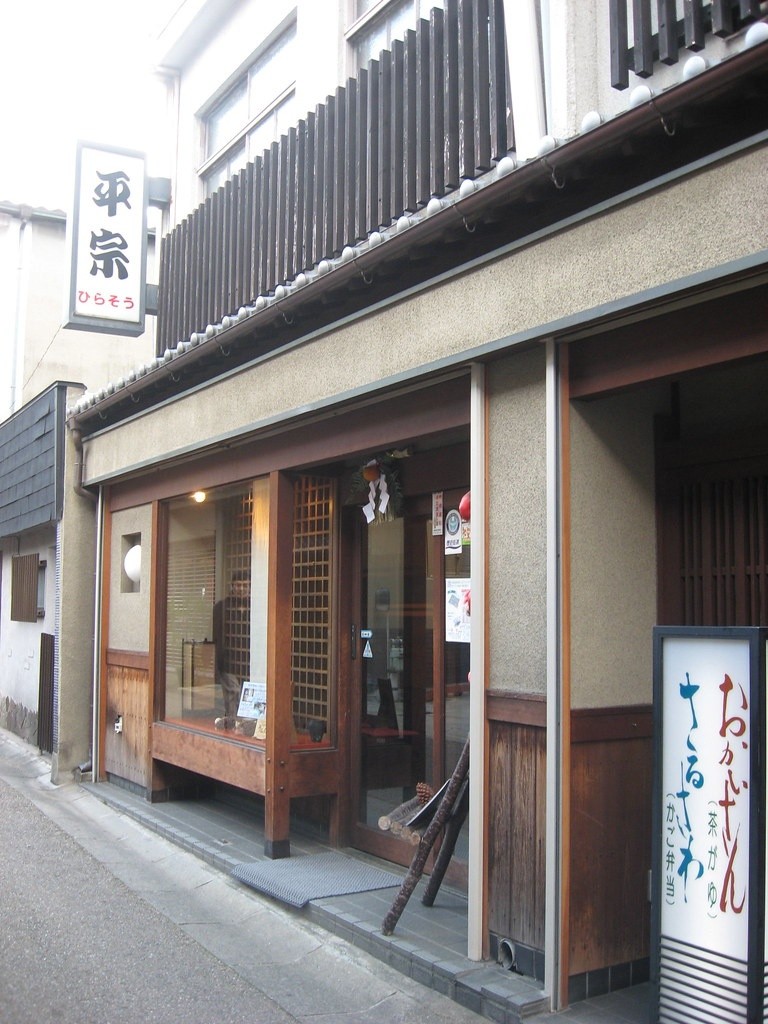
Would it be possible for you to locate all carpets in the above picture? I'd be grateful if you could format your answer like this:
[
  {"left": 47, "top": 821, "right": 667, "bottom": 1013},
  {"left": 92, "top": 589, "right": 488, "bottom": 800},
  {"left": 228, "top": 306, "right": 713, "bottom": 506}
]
[{"left": 232, "top": 850, "right": 403, "bottom": 908}]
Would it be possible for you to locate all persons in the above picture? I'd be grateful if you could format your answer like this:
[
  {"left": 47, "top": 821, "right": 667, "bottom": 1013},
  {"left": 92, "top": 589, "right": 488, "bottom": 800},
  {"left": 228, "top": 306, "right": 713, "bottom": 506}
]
[{"left": 213, "top": 571, "right": 250, "bottom": 729}]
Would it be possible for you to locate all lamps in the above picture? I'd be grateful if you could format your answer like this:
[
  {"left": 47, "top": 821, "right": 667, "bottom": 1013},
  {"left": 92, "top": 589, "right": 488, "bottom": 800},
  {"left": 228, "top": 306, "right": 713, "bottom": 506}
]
[
  {"left": 122, "top": 545, "right": 142, "bottom": 585},
  {"left": 181, "top": 638, "right": 219, "bottom": 721}
]
